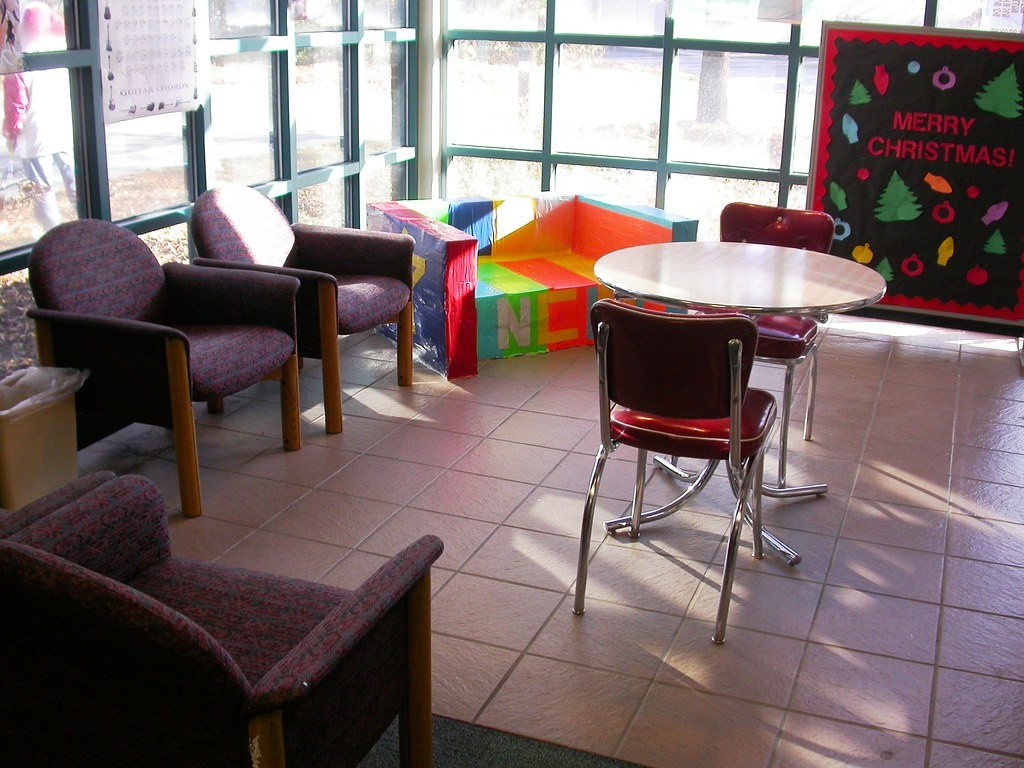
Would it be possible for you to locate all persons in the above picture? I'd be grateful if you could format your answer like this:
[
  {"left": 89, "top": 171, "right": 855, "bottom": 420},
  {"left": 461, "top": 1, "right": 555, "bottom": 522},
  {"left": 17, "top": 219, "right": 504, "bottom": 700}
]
[{"left": 2, "top": 0, "right": 78, "bottom": 229}]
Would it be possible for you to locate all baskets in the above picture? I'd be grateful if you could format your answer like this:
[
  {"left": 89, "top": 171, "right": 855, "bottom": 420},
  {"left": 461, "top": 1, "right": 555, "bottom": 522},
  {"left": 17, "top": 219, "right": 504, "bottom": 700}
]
[{"left": 1, "top": 179, "right": 36, "bottom": 227}]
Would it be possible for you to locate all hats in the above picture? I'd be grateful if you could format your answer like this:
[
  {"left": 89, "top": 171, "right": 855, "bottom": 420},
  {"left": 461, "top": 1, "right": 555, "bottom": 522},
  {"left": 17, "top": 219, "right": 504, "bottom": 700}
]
[{"left": 19, "top": 4, "right": 65, "bottom": 39}]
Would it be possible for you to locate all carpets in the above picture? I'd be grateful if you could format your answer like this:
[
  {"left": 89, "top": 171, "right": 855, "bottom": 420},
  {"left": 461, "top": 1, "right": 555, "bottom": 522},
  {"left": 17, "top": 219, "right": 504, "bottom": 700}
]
[{"left": 357, "top": 713, "right": 646, "bottom": 768}]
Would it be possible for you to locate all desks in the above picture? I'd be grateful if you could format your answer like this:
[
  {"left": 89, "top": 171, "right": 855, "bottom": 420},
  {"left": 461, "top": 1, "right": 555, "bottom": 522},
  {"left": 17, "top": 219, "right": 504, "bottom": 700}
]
[{"left": 592, "top": 240, "right": 886, "bottom": 565}]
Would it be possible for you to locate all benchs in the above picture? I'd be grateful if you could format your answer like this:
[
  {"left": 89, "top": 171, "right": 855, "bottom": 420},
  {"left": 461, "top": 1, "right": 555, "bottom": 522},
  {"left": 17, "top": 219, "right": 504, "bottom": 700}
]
[{"left": 366, "top": 190, "right": 699, "bottom": 380}]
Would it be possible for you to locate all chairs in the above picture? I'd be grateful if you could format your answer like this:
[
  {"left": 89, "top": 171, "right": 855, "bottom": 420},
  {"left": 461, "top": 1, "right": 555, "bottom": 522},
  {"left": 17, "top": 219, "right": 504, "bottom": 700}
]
[
  {"left": 191, "top": 183, "right": 415, "bottom": 434},
  {"left": 671, "top": 202, "right": 836, "bottom": 468},
  {"left": 0, "top": 469, "right": 445, "bottom": 768},
  {"left": 572, "top": 295, "right": 777, "bottom": 644},
  {"left": 27, "top": 219, "right": 301, "bottom": 518}
]
